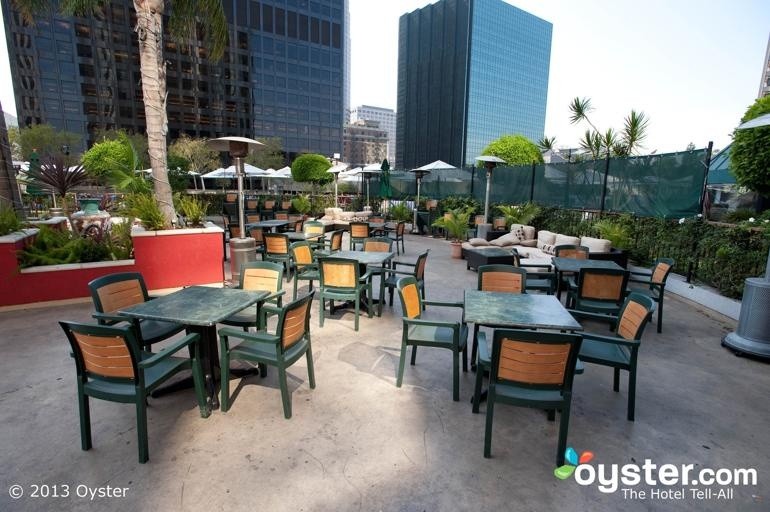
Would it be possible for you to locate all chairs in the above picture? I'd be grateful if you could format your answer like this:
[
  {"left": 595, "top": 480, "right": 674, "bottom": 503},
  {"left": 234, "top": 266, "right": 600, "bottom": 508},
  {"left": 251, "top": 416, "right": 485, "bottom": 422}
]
[{"left": 63, "top": 184, "right": 669, "bottom": 462}]
